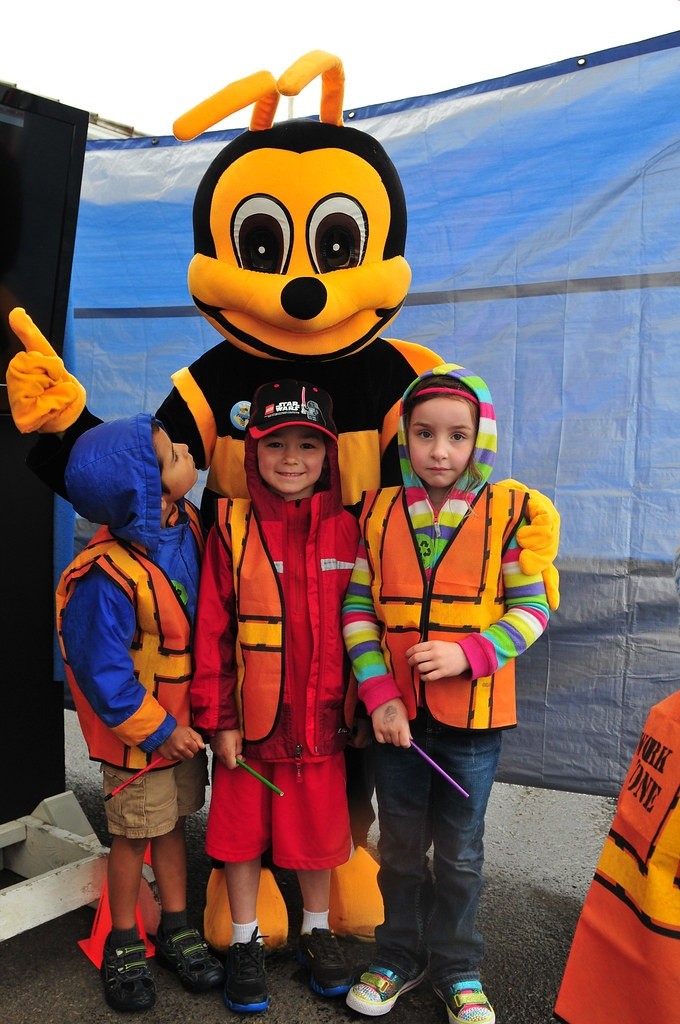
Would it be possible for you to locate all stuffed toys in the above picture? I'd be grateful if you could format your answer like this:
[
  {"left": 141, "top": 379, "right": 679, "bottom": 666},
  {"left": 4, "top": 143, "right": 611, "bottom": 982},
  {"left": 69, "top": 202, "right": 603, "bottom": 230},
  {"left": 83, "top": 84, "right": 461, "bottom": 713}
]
[{"left": 6, "top": 53, "right": 560, "bottom": 946}]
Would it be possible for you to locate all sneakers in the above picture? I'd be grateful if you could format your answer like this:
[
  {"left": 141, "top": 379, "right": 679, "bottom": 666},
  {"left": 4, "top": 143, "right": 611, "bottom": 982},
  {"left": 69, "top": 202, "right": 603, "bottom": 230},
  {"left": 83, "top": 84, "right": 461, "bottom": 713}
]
[
  {"left": 154, "top": 920, "right": 228, "bottom": 994},
  {"left": 223, "top": 940, "right": 270, "bottom": 1014},
  {"left": 297, "top": 927, "right": 356, "bottom": 998},
  {"left": 98, "top": 939, "right": 157, "bottom": 1014}
]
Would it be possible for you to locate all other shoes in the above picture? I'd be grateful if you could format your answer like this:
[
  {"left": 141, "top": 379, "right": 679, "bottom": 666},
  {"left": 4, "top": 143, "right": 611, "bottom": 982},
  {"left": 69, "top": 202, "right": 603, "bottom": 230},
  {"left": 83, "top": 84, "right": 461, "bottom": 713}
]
[
  {"left": 345, "top": 949, "right": 426, "bottom": 1016},
  {"left": 430, "top": 970, "right": 496, "bottom": 1024}
]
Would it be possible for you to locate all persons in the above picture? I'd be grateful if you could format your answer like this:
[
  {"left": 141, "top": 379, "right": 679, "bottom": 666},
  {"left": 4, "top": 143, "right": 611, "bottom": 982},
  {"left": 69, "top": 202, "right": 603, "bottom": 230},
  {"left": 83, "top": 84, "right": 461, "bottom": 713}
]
[
  {"left": 554, "top": 689, "right": 680, "bottom": 1024},
  {"left": 54, "top": 413, "right": 226, "bottom": 1013},
  {"left": 191, "top": 380, "right": 362, "bottom": 1013},
  {"left": 341, "top": 362, "right": 550, "bottom": 1023}
]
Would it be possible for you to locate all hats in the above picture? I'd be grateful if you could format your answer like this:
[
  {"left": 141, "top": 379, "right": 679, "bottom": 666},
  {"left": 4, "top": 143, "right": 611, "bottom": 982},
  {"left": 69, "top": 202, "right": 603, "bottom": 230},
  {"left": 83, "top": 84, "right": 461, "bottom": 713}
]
[{"left": 245, "top": 378, "right": 339, "bottom": 441}]
